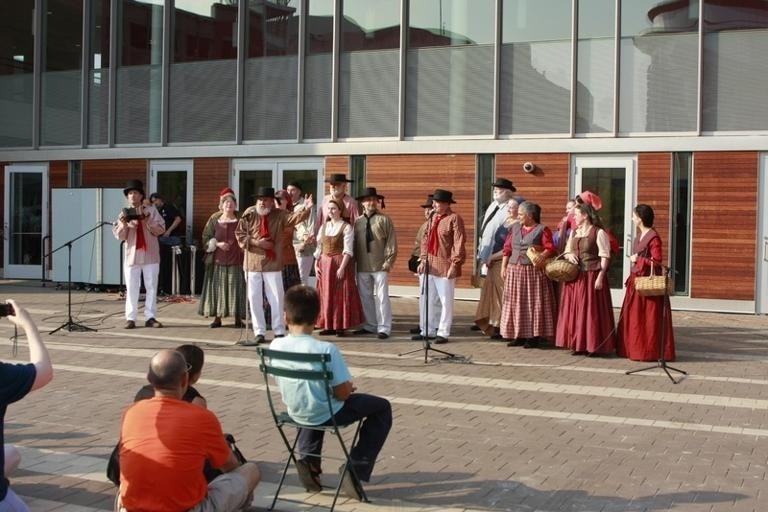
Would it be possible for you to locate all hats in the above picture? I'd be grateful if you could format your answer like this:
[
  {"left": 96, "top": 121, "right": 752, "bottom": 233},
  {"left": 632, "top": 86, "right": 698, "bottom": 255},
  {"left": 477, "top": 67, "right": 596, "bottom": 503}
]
[
  {"left": 124, "top": 179, "right": 146, "bottom": 197},
  {"left": 420, "top": 194, "right": 433, "bottom": 208},
  {"left": 251, "top": 187, "right": 280, "bottom": 199},
  {"left": 219, "top": 187, "right": 235, "bottom": 199},
  {"left": 323, "top": 173, "right": 355, "bottom": 183},
  {"left": 355, "top": 187, "right": 384, "bottom": 200},
  {"left": 490, "top": 176, "right": 516, "bottom": 192},
  {"left": 428, "top": 189, "right": 457, "bottom": 204}
]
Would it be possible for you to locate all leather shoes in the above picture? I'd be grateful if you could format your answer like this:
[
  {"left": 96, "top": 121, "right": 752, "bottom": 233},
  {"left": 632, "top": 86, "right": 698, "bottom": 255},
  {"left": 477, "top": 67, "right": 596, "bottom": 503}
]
[
  {"left": 314, "top": 325, "right": 543, "bottom": 349},
  {"left": 255, "top": 335, "right": 265, "bottom": 343}
]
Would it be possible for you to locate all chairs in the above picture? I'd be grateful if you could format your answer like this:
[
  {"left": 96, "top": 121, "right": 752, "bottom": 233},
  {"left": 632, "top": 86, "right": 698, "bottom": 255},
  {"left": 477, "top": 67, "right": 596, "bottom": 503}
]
[{"left": 256, "top": 347, "right": 372, "bottom": 512}]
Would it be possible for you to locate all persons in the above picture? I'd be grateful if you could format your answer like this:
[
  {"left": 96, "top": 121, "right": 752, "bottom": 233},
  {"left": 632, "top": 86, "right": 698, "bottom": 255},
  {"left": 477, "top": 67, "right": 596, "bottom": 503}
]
[
  {"left": 106, "top": 344, "right": 243, "bottom": 492},
  {"left": 408, "top": 194, "right": 439, "bottom": 335},
  {"left": 617, "top": 204, "right": 676, "bottom": 362},
  {"left": 149, "top": 193, "right": 182, "bottom": 292},
  {"left": 416, "top": 191, "right": 467, "bottom": 343},
  {"left": 113, "top": 348, "right": 262, "bottom": 511},
  {"left": 112, "top": 189, "right": 164, "bottom": 329},
  {"left": 469, "top": 177, "right": 616, "bottom": 355},
  {"left": 198, "top": 173, "right": 399, "bottom": 345},
  {"left": 268, "top": 284, "right": 392, "bottom": 502},
  {"left": 0, "top": 298, "right": 54, "bottom": 512}
]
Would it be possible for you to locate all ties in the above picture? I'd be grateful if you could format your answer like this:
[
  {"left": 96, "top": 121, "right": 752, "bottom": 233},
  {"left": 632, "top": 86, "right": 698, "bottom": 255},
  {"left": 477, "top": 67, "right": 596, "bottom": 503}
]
[{"left": 479, "top": 206, "right": 499, "bottom": 239}]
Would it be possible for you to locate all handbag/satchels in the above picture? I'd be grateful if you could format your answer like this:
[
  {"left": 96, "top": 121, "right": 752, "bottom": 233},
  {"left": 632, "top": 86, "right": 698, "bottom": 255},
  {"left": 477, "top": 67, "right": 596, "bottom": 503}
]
[{"left": 409, "top": 247, "right": 419, "bottom": 273}]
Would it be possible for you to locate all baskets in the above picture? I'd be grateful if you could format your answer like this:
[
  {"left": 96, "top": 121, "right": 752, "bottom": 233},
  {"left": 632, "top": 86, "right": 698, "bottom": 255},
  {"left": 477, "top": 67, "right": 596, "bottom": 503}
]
[
  {"left": 471, "top": 261, "right": 488, "bottom": 288},
  {"left": 526, "top": 244, "right": 560, "bottom": 270},
  {"left": 634, "top": 260, "right": 673, "bottom": 296},
  {"left": 544, "top": 252, "right": 580, "bottom": 282}
]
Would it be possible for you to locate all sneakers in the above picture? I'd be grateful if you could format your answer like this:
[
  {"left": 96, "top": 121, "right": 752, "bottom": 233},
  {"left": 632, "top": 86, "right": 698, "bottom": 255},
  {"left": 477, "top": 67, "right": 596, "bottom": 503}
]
[
  {"left": 124, "top": 320, "right": 135, "bottom": 329},
  {"left": 145, "top": 318, "right": 163, "bottom": 328},
  {"left": 235, "top": 320, "right": 244, "bottom": 329},
  {"left": 210, "top": 321, "right": 222, "bottom": 329}
]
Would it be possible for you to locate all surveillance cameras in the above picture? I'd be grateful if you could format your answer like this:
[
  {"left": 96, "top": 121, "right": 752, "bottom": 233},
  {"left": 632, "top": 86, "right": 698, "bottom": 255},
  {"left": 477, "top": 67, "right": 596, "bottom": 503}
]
[{"left": 523, "top": 162, "right": 534, "bottom": 173}]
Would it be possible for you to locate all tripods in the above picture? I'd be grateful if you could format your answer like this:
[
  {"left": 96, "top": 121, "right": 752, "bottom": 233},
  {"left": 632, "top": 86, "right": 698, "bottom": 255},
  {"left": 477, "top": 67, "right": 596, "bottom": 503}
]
[
  {"left": 625, "top": 258, "right": 687, "bottom": 383},
  {"left": 398, "top": 222, "right": 454, "bottom": 364},
  {"left": 43, "top": 223, "right": 103, "bottom": 334}
]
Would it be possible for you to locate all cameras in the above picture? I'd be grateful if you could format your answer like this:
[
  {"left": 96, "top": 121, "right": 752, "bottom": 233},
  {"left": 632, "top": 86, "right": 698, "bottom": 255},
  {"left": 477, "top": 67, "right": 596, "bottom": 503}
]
[{"left": 0, "top": 304, "right": 13, "bottom": 317}]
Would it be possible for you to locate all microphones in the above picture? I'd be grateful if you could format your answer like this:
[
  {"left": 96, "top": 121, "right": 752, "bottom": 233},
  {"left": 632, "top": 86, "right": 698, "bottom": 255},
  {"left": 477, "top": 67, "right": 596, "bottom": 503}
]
[
  {"left": 426, "top": 209, "right": 436, "bottom": 221},
  {"left": 242, "top": 209, "right": 256, "bottom": 221},
  {"left": 637, "top": 247, "right": 647, "bottom": 257},
  {"left": 103, "top": 221, "right": 118, "bottom": 226}
]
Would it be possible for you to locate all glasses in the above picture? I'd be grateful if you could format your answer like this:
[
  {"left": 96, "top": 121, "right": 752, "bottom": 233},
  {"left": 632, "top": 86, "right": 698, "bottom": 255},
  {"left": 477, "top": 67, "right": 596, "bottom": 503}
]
[{"left": 185, "top": 361, "right": 193, "bottom": 375}]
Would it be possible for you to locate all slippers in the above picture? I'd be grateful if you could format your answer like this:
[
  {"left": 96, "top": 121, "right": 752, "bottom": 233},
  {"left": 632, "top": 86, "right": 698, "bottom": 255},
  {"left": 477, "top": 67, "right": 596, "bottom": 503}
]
[
  {"left": 296, "top": 457, "right": 323, "bottom": 494},
  {"left": 338, "top": 464, "right": 363, "bottom": 501}
]
